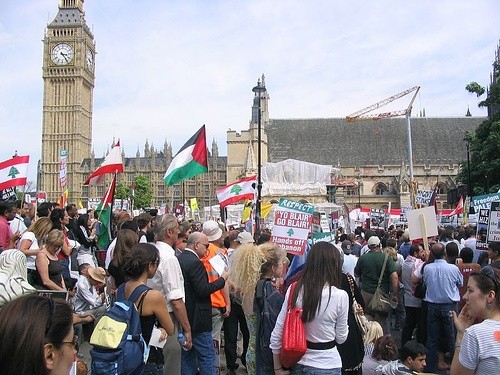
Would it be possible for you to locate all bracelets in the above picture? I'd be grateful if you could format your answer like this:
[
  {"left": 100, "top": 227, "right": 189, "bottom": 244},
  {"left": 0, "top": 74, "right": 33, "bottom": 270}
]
[{"left": 454, "top": 346, "right": 460, "bottom": 348}]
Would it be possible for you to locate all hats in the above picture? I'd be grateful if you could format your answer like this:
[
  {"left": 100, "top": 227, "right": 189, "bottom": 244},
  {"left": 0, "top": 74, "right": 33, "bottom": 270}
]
[
  {"left": 368, "top": 236, "right": 380, "bottom": 248},
  {"left": 341, "top": 240, "right": 352, "bottom": 254},
  {"left": 201, "top": 221, "right": 222, "bottom": 242},
  {"left": 87, "top": 266, "right": 106, "bottom": 284},
  {"left": 234, "top": 232, "right": 255, "bottom": 244}
]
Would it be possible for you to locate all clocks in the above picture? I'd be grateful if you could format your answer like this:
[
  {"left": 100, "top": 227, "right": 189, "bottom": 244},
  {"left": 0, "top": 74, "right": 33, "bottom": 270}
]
[
  {"left": 51, "top": 42, "right": 74, "bottom": 65},
  {"left": 87, "top": 49, "right": 93, "bottom": 69}
]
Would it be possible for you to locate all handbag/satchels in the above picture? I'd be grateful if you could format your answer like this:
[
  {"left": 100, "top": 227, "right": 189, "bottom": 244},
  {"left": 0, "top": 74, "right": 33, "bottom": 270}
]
[
  {"left": 280, "top": 281, "right": 307, "bottom": 370},
  {"left": 367, "top": 287, "right": 392, "bottom": 317}
]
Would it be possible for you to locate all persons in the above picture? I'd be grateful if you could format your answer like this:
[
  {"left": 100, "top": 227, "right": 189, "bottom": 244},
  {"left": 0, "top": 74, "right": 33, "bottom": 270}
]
[{"left": 0, "top": 200, "right": 500, "bottom": 375}]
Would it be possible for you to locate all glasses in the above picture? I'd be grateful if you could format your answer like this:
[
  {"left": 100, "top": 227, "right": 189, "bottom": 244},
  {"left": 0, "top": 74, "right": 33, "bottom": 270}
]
[
  {"left": 60, "top": 335, "right": 79, "bottom": 346},
  {"left": 480, "top": 265, "right": 499, "bottom": 290},
  {"left": 200, "top": 242, "right": 209, "bottom": 249}
]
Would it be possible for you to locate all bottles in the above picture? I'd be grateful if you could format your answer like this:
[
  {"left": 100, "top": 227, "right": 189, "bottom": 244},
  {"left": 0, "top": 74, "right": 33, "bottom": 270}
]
[{"left": 178, "top": 334, "right": 189, "bottom": 351}]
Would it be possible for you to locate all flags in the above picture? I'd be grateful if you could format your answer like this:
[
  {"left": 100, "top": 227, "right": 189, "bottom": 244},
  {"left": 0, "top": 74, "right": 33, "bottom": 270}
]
[
  {"left": 162, "top": 124, "right": 209, "bottom": 188},
  {"left": 83, "top": 141, "right": 123, "bottom": 251},
  {"left": 215, "top": 174, "right": 257, "bottom": 209},
  {"left": 0, "top": 156, "right": 29, "bottom": 192},
  {"left": 447, "top": 194, "right": 464, "bottom": 219}
]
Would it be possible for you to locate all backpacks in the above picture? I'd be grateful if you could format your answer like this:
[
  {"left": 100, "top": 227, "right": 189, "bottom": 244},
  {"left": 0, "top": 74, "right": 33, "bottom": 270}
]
[{"left": 90, "top": 283, "right": 153, "bottom": 375}]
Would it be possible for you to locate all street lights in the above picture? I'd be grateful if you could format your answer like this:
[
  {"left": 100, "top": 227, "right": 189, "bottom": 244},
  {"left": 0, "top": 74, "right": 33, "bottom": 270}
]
[{"left": 251, "top": 78, "right": 266, "bottom": 230}]
